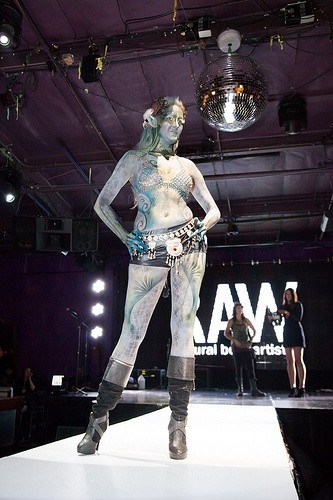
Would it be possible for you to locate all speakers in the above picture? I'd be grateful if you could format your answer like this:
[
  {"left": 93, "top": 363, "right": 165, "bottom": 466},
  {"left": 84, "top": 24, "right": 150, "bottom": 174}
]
[
  {"left": 160, "top": 368, "right": 209, "bottom": 391},
  {"left": 11, "top": 215, "right": 99, "bottom": 253}
]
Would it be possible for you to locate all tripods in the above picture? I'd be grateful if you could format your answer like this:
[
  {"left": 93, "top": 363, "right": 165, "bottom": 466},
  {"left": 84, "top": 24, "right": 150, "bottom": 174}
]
[{"left": 64, "top": 311, "right": 97, "bottom": 395}]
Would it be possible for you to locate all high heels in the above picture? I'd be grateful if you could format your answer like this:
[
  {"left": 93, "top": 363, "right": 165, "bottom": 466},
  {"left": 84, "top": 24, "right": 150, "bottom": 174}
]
[
  {"left": 294, "top": 388, "right": 306, "bottom": 397},
  {"left": 288, "top": 388, "right": 297, "bottom": 397}
]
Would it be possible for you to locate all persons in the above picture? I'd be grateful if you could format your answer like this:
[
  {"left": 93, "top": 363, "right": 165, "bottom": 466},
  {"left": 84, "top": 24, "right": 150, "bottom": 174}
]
[
  {"left": 76, "top": 95, "right": 221, "bottom": 460},
  {"left": 4, "top": 364, "right": 37, "bottom": 438},
  {"left": 268, "top": 288, "right": 307, "bottom": 398},
  {"left": 224, "top": 303, "right": 266, "bottom": 396}
]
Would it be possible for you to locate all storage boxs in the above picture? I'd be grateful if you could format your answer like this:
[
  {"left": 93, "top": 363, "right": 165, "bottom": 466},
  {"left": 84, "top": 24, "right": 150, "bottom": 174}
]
[{"left": 138, "top": 369, "right": 166, "bottom": 389}]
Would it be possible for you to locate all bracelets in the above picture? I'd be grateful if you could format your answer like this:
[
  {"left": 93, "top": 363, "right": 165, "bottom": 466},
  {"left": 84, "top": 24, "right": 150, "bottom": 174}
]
[{"left": 285, "top": 311, "right": 287, "bottom": 315}]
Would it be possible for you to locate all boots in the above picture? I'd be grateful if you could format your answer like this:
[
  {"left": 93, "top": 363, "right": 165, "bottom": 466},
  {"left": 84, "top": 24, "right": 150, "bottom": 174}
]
[
  {"left": 77, "top": 357, "right": 134, "bottom": 454},
  {"left": 236, "top": 378, "right": 243, "bottom": 396},
  {"left": 166, "top": 356, "right": 195, "bottom": 459},
  {"left": 249, "top": 377, "right": 266, "bottom": 396}
]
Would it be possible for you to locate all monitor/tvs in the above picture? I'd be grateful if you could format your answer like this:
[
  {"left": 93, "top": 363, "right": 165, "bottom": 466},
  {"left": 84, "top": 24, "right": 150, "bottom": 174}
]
[{"left": 51, "top": 374, "right": 64, "bottom": 386}]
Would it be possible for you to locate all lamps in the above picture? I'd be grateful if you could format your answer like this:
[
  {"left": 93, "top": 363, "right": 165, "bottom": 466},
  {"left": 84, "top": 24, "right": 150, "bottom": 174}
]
[
  {"left": 278, "top": 95, "right": 308, "bottom": 134},
  {"left": 0, "top": 15, "right": 15, "bottom": 48},
  {"left": 80, "top": 55, "right": 99, "bottom": 83},
  {"left": 0, "top": 184, "right": 15, "bottom": 203},
  {"left": 0, "top": 88, "right": 16, "bottom": 108},
  {"left": 195, "top": 28, "right": 269, "bottom": 133},
  {"left": 227, "top": 222, "right": 239, "bottom": 237}
]
